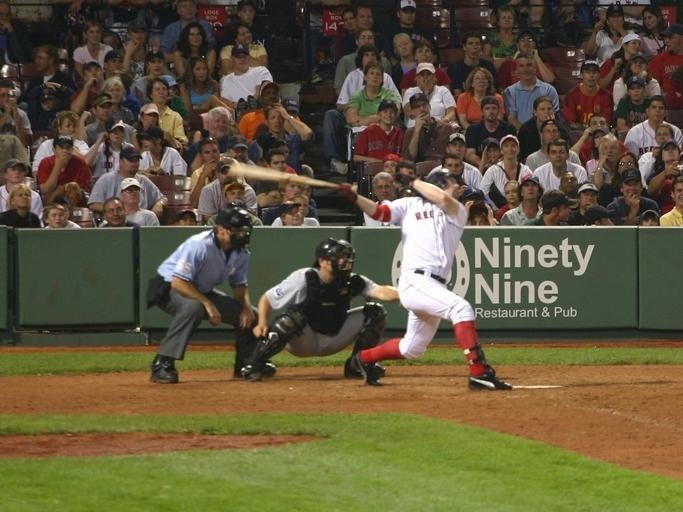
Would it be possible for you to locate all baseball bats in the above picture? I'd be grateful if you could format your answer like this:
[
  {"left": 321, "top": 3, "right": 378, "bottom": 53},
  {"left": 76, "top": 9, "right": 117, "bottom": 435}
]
[{"left": 227, "top": 164, "right": 339, "bottom": 186}]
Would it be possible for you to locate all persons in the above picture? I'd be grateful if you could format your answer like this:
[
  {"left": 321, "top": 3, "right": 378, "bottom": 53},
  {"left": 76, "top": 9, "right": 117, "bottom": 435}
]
[
  {"left": 3, "top": 0, "right": 683, "bottom": 228},
  {"left": 331, "top": 170, "right": 513, "bottom": 392},
  {"left": 241, "top": 237, "right": 402, "bottom": 382},
  {"left": 144, "top": 205, "right": 264, "bottom": 384}
]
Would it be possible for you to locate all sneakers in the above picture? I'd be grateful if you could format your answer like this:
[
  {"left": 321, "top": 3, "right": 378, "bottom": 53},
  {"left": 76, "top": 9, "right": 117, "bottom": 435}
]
[
  {"left": 468, "top": 376, "right": 512, "bottom": 389},
  {"left": 234, "top": 362, "right": 276, "bottom": 378},
  {"left": 343, "top": 348, "right": 387, "bottom": 388},
  {"left": 151, "top": 357, "right": 177, "bottom": 383},
  {"left": 302, "top": 71, "right": 333, "bottom": 85},
  {"left": 330, "top": 160, "right": 347, "bottom": 176}
]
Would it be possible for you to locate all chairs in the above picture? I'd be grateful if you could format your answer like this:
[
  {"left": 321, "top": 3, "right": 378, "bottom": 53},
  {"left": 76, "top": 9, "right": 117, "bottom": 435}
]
[
  {"left": 537, "top": 46, "right": 587, "bottom": 97},
  {"left": 416, "top": 0, "right": 493, "bottom": 65}
]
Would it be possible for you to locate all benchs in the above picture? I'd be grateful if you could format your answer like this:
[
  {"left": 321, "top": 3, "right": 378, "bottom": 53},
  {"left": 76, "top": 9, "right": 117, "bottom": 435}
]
[
  {"left": 66, "top": 175, "right": 203, "bottom": 227},
  {"left": 355, "top": 156, "right": 444, "bottom": 206}
]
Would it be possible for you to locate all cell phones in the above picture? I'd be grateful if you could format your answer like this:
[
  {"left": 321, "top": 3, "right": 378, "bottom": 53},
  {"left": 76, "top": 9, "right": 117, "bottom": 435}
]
[{"left": 9, "top": 90, "right": 17, "bottom": 101}]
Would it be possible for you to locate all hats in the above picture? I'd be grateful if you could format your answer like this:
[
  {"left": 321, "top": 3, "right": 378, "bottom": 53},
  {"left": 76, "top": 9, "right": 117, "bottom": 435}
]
[
  {"left": 53, "top": 134, "right": 73, "bottom": 149},
  {"left": 519, "top": 167, "right": 660, "bottom": 224},
  {"left": 396, "top": 0, "right": 417, "bottom": 11},
  {"left": 446, "top": 97, "right": 519, "bottom": 151},
  {"left": 582, "top": 3, "right": 683, "bottom": 88},
  {"left": 378, "top": 61, "right": 436, "bottom": 112},
  {"left": 4, "top": 159, "right": 27, "bottom": 172},
  {"left": 40, "top": 87, "right": 55, "bottom": 103},
  {"left": 81, "top": 18, "right": 165, "bottom": 70},
  {"left": 462, "top": 188, "right": 488, "bottom": 204},
  {"left": 282, "top": 98, "right": 299, "bottom": 113},
  {"left": 92, "top": 92, "right": 161, "bottom": 190},
  {"left": 279, "top": 201, "right": 302, "bottom": 213},
  {"left": 176, "top": 209, "right": 196, "bottom": 220},
  {"left": 231, "top": 43, "right": 249, "bottom": 56},
  {"left": 217, "top": 135, "right": 248, "bottom": 196}
]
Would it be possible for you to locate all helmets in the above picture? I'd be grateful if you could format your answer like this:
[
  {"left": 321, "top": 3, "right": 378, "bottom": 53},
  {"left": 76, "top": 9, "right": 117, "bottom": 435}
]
[
  {"left": 217, "top": 206, "right": 253, "bottom": 251},
  {"left": 425, "top": 165, "right": 459, "bottom": 187},
  {"left": 315, "top": 236, "right": 355, "bottom": 293}
]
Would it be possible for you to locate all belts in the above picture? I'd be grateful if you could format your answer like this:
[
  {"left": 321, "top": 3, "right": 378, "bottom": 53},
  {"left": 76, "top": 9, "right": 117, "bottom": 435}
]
[{"left": 415, "top": 270, "right": 446, "bottom": 284}]
